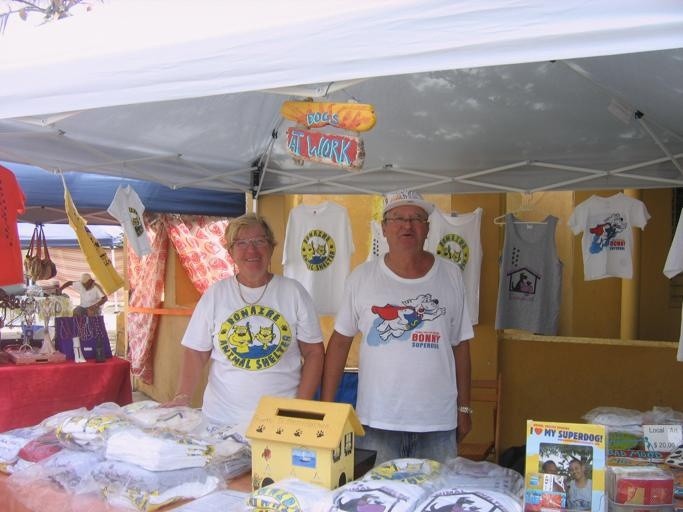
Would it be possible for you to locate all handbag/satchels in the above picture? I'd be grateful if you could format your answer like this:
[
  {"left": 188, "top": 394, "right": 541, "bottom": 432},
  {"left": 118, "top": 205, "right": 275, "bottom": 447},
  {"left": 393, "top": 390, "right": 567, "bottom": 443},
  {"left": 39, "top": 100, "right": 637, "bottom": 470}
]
[{"left": 25, "top": 225, "right": 57, "bottom": 281}]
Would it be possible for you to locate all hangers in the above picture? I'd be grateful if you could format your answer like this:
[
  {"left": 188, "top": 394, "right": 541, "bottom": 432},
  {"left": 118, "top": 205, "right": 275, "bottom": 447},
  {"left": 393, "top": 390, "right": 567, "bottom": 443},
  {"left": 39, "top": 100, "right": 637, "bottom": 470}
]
[
  {"left": 53, "top": 168, "right": 67, "bottom": 195},
  {"left": 493, "top": 192, "right": 562, "bottom": 228}
]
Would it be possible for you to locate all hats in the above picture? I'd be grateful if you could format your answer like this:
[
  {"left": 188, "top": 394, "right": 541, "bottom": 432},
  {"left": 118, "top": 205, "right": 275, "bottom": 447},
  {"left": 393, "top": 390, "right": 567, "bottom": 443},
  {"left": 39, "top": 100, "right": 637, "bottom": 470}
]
[
  {"left": 381, "top": 189, "right": 435, "bottom": 215},
  {"left": 79, "top": 273, "right": 91, "bottom": 284}
]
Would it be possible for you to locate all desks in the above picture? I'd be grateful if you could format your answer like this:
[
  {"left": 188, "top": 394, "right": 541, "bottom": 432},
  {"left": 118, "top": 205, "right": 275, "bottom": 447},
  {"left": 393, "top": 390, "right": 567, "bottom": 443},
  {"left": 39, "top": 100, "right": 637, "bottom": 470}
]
[
  {"left": 0, "top": 340, "right": 133, "bottom": 434},
  {"left": 0, "top": 448, "right": 252, "bottom": 512}
]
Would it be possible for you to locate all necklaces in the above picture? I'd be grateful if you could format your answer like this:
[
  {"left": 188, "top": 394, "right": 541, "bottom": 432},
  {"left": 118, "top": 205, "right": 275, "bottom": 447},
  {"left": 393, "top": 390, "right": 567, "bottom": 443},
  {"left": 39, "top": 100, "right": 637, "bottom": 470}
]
[{"left": 237, "top": 273, "right": 269, "bottom": 304}]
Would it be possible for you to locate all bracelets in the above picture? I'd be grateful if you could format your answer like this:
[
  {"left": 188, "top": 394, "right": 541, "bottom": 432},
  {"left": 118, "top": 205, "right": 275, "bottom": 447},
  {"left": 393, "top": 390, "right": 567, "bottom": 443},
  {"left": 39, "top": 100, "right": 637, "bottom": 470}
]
[{"left": 173, "top": 393, "right": 193, "bottom": 403}]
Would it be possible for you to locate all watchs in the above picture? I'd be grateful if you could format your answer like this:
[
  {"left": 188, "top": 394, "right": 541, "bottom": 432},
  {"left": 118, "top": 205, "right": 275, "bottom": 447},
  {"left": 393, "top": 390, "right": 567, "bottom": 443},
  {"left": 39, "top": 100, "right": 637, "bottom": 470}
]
[{"left": 457, "top": 405, "right": 473, "bottom": 415}]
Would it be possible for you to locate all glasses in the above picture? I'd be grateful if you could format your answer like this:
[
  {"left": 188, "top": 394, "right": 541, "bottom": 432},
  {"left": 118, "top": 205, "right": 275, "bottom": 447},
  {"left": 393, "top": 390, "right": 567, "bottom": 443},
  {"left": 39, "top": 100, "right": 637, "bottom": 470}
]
[
  {"left": 235, "top": 236, "right": 268, "bottom": 249},
  {"left": 386, "top": 216, "right": 429, "bottom": 224}
]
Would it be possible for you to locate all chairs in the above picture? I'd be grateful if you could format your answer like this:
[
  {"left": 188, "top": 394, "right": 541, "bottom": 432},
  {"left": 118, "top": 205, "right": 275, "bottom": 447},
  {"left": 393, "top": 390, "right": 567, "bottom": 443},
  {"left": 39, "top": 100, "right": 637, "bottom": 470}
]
[{"left": 459, "top": 371, "right": 503, "bottom": 465}]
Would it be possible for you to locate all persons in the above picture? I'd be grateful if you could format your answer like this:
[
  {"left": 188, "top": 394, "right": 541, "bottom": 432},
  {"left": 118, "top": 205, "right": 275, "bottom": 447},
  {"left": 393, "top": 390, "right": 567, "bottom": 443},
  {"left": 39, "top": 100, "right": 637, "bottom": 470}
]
[
  {"left": 60, "top": 273, "right": 108, "bottom": 315},
  {"left": 320, "top": 189, "right": 476, "bottom": 467},
  {"left": 543, "top": 461, "right": 557, "bottom": 474},
  {"left": 157, "top": 212, "right": 326, "bottom": 442},
  {"left": 565, "top": 460, "right": 592, "bottom": 512}
]
